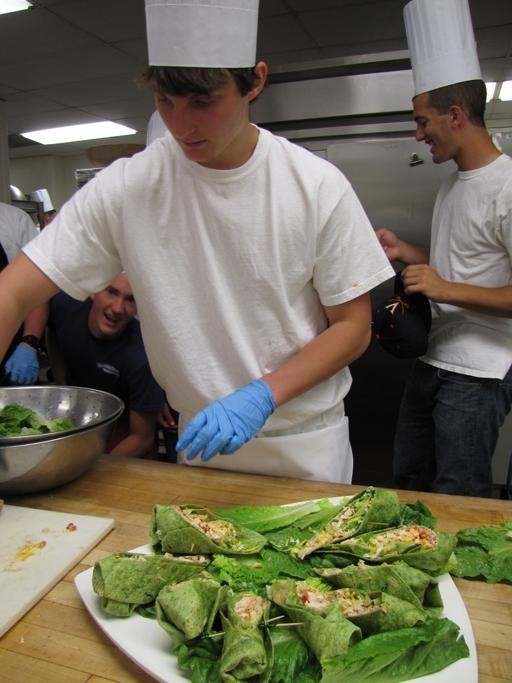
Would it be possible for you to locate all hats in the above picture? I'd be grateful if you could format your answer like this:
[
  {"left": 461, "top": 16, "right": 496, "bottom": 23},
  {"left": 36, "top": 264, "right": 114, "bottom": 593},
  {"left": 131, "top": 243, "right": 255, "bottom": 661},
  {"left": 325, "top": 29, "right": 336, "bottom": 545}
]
[
  {"left": 403, "top": 0, "right": 483, "bottom": 98},
  {"left": 144, "top": 0, "right": 258, "bottom": 70},
  {"left": 372, "top": 271, "right": 432, "bottom": 359},
  {"left": 33, "top": 189, "right": 54, "bottom": 213}
]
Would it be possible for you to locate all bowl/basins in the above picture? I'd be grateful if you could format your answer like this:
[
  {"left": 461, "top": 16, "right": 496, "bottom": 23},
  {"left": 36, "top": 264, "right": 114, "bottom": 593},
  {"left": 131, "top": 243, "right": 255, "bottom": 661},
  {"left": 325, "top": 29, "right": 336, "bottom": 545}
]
[{"left": 0, "top": 384, "right": 126, "bottom": 497}]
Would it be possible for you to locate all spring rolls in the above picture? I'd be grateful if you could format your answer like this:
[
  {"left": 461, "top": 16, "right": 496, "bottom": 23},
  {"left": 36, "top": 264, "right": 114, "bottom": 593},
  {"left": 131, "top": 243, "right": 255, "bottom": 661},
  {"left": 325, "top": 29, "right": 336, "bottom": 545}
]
[{"left": 93, "top": 487, "right": 456, "bottom": 682}]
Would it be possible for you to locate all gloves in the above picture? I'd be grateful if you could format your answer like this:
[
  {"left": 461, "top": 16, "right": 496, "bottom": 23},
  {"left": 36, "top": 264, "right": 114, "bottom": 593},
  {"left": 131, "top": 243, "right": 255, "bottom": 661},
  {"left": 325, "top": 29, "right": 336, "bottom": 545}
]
[
  {"left": 4, "top": 346, "right": 40, "bottom": 386},
  {"left": 177, "top": 380, "right": 276, "bottom": 462}
]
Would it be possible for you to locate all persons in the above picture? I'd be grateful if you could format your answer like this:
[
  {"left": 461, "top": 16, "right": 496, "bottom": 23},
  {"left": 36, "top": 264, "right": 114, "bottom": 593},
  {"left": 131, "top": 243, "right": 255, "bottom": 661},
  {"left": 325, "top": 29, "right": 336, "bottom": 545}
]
[
  {"left": 0, "top": 188, "right": 179, "bottom": 460},
  {"left": 1, "top": 0, "right": 395, "bottom": 486},
  {"left": 375, "top": 79, "right": 511, "bottom": 498}
]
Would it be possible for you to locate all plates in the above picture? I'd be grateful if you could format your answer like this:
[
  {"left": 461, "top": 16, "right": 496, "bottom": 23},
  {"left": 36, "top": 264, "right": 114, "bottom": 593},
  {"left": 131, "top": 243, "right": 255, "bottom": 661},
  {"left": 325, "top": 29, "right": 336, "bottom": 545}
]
[{"left": 73, "top": 492, "right": 481, "bottom": 683}]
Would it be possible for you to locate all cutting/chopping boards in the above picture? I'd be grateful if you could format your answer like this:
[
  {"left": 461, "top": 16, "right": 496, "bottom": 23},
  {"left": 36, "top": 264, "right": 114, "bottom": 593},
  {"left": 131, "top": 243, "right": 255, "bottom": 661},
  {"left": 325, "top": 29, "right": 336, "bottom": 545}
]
[{"left": 1, "top": 502, "right": 115, "bottom": 637}]
